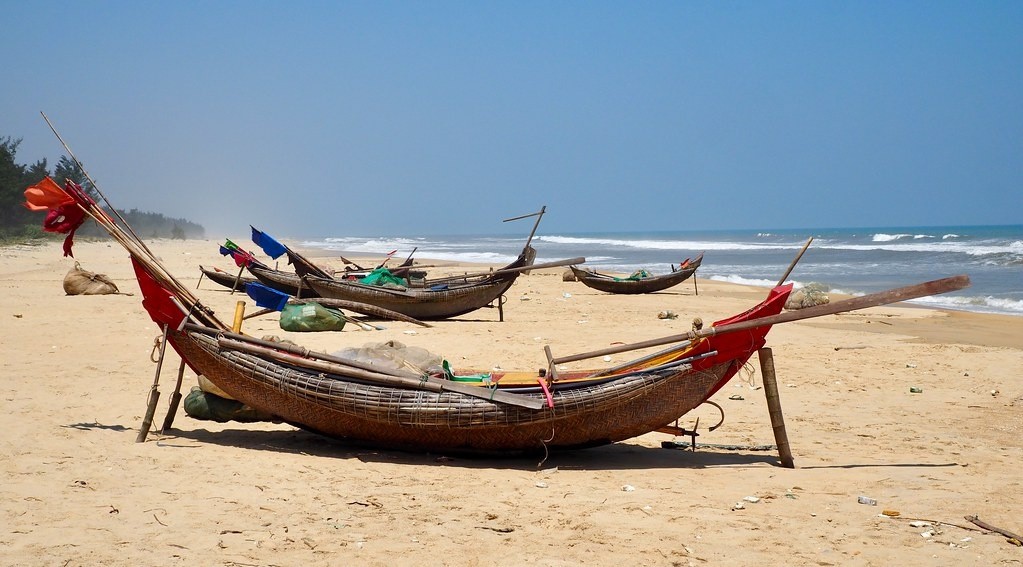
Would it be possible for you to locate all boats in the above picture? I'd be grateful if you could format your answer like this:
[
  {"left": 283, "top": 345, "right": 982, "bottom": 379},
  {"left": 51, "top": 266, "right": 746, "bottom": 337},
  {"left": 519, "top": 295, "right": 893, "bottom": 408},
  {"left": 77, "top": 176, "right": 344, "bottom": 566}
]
[
  {"left": 199, "top": 248, "right": 418, "bottom": 294},
  {"left": 22, "top": 109, "right": 814, "bottom": 457},
  {"left": 218, "top": 236, "right": 490, "bottom": 299},
  {"left": 345, "top": 266, "right": 428, "bottom": 279},
  {"left": 568, "top": 252, "right": 704, "bottom": 294},
  {"left": 247, "top": 205, "right": 547, "bottom": 322}
]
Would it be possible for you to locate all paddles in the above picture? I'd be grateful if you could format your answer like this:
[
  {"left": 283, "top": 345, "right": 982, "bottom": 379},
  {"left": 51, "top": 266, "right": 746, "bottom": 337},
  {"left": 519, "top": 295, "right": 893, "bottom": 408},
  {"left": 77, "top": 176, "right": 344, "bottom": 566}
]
[
  {"left": 177, "top": 316, "right": 545, "bottom": 409},
  {"left": 335, "top": 262, "right": 461, "bottom": 274},
  {"left": 424, "top": 257, "right": 585, "bottom": 289},
  {"left": 544, "top": 274, "right": 973, "bottom": 382}
]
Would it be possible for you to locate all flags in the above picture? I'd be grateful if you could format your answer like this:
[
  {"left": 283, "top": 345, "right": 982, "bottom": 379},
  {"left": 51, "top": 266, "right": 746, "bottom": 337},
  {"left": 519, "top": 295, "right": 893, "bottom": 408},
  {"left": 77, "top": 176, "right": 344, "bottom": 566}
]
[
  {"left": 218, "top": 229, "right": 290, "bottom": 311},
  {"left": 22, "top": 177, "right": 95, "bottom": 257}
]
